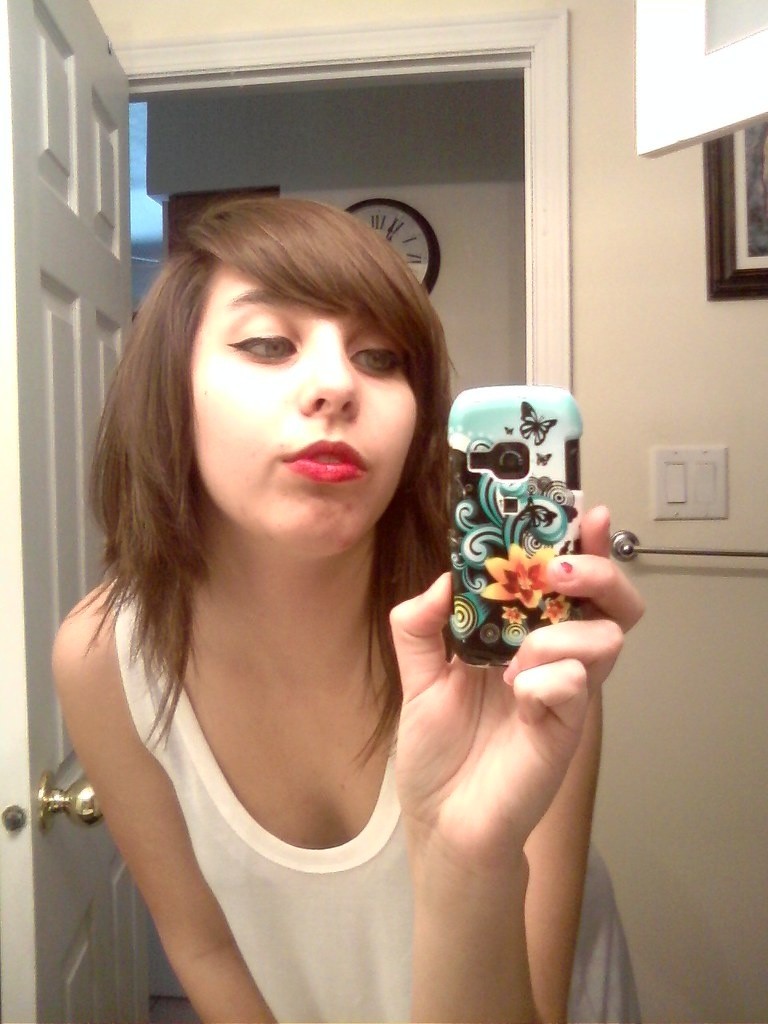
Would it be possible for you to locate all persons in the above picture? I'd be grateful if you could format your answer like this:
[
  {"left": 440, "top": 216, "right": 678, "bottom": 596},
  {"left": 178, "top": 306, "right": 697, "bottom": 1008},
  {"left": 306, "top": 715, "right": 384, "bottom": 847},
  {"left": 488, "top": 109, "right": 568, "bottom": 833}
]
[{"left": 52, "top": 198, "right": 645, "bottom": 1024}]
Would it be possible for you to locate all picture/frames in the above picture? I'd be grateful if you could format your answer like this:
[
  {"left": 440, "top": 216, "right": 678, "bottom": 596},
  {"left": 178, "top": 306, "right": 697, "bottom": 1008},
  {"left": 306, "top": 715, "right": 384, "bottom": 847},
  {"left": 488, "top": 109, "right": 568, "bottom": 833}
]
[{"left": 703, "top": 119, "right": 768, "bottom": 300}]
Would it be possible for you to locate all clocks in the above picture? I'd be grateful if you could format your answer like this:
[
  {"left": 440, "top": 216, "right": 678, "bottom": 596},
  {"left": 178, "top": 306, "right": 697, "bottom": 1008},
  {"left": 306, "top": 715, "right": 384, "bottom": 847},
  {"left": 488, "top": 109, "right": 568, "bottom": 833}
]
[{"left": 342, "top": 198, "right": 441, "bottom": 297}]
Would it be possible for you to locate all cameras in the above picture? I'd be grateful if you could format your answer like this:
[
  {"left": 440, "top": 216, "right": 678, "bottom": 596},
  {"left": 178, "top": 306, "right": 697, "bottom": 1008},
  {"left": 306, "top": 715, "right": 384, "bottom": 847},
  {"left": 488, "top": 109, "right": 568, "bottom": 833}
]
[{"left": 445, "top": 386, "right": 582, "bottom": 667}]
